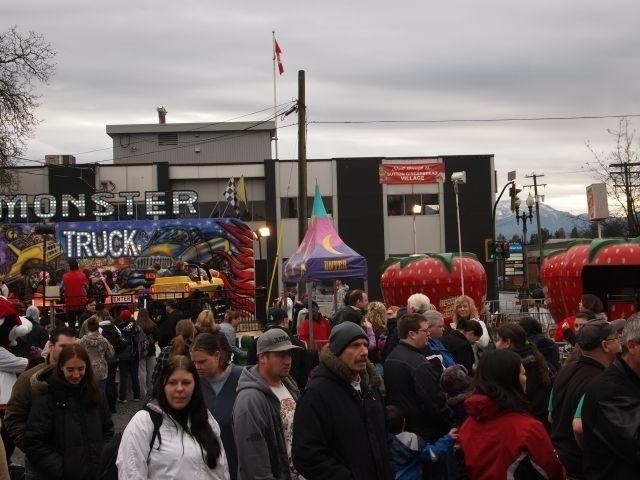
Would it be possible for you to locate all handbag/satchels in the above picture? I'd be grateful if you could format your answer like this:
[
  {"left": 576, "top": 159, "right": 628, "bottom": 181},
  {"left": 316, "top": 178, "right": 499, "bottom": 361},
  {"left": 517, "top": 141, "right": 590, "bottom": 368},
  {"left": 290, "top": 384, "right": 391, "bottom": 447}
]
[
  {"left": 96, "top": 406, "right": 158, "bottom": 480},
  {"left": 154, "top": 343, "right": 162, "bottom": 359}
]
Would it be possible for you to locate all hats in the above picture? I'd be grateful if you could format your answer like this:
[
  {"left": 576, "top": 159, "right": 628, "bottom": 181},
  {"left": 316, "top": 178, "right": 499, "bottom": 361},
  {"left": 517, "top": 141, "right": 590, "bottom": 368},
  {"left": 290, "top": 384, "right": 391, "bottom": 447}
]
[
  {"left": 257, "top": 328, "right": 303, "bottom": 351},
  {"left": 332, "top": 277, "right": 342, "bottom": 281},
  {"left": 303, "top": 299, "right": 317, "bottom": 309},
  {"left": 267, "top": 307, "right": 288, "bottom": 322},
  {"left": 339, "top": 305, "right": 362, "bottom": 325},
  {"left": 574, "top": 317, "right": 627, "bottom": 352},
  {"left": 120, "top": 308, "right": 132, "bottom": 321},
  {"left": 328, "top": 321, "right": 370, "bottom": 357},
  {"left": 440, "top": 365, "right": 474, "bottom": 395}
]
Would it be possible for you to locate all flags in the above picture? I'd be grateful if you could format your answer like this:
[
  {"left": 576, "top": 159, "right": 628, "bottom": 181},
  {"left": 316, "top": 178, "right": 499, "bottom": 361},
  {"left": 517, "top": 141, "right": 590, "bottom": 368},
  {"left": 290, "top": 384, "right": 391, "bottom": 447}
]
[
  {"left": 235, "top": 176, "right": 248, "bottom": 211},
  {"left": 223, "top": 178, "right": 240, "bottom": 215},
  {"left": 275, "top": 40, "right": 284, "bottom": 75}
]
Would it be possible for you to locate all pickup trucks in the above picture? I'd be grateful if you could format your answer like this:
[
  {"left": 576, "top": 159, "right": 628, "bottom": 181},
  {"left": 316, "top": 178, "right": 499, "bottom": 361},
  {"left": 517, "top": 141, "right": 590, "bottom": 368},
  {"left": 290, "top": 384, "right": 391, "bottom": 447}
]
[{"left": 130, "top": 225, "right": 230, "bottom": 278}]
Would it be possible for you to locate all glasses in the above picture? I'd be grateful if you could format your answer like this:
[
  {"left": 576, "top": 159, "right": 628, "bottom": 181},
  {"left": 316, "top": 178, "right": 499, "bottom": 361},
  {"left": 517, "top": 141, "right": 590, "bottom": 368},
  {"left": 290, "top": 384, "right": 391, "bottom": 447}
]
[
  {"left": 415, "top": 327, "right": 431, "bottom": 335},
  {"left": 348, "top": 340, "right": 369, "bottom": 351},
  {"left": 605, "top": 333, "right": 625, "bottom": 345},
  {"left": 273, "top": 349, "right": 293, "bottom": 358}
]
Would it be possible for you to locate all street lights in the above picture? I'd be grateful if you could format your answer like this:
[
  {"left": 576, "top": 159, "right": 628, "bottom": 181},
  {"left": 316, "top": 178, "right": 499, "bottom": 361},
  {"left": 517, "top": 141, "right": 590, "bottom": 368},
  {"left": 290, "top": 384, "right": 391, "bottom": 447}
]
[
  {"left": 451, "top": 170, "right": 467, "bottom": 297},
  {"left": 513, "top": 192, "right": 534, "bottom": 312},
  {"left": 411, "top": 203, "right": 422, "bottom": 252},
  {"left": 511, "top": 193, "right": 535, "bottom": 301},
  {"left": 446, "top": 168, "right": 468, "bottom": 296}
]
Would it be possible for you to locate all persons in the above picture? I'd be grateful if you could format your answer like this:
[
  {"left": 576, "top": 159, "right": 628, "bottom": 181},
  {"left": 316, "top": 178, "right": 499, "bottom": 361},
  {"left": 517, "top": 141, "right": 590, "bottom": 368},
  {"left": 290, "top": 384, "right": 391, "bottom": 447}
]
[
  {"left": 99, "top": 270, "right": 117, "bottom": 297},
  {"left": 59, "top": 260, "right": 88, "bottom": 320}
]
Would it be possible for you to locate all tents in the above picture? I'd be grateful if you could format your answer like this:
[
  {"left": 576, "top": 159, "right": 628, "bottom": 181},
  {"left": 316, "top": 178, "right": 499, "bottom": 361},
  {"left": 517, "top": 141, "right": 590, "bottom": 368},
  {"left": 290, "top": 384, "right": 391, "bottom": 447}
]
[{"left": 282, "top": 177, "right": 369, "bottom": 350}]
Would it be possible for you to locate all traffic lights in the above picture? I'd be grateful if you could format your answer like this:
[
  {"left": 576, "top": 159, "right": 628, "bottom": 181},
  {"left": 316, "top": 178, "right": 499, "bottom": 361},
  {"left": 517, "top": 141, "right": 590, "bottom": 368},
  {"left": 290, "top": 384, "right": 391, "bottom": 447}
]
[{"left": 489, "top": 240, "right": 510, "bottom": 260}]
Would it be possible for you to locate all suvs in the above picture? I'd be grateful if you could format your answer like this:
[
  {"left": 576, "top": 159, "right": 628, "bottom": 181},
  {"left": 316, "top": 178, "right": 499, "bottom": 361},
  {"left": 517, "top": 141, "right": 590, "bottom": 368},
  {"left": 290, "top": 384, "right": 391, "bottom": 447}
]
[
  {"left": 149, "top": 263, "right": 224, "bottom": 305},
  {"left": 0, "top": 236, "right": 64, "bottom": 295}
]
